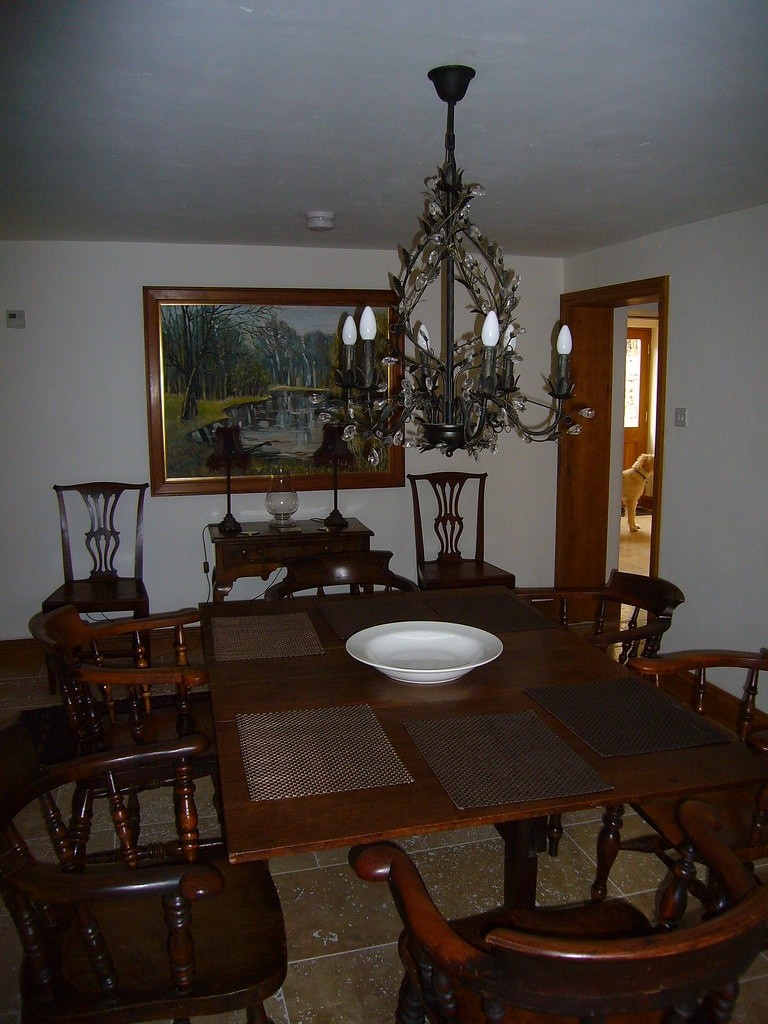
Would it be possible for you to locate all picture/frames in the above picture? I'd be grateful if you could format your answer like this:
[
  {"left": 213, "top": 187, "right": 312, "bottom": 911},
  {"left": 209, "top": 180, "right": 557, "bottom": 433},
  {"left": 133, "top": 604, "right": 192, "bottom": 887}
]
[{"left": 141, "top": 286, "right": 406, "bottom": 497}]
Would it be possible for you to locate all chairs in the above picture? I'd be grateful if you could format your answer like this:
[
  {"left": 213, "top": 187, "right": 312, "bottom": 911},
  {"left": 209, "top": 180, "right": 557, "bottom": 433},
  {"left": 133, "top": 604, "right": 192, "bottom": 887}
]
[
  {"left": 0, "top": 735, "right": 289, "bottom": 1024},
  {"left": 591, "top": 649, "right": 768, "bottom": 928},
  {"left": 42, "top": 483, "right": 151, "bottom": 696},
  {"left": 264, "top": 549, "right": 420, "bottom": 603},
  {"left": 406, "top": 471, "right": 517, "bottom": 593},
  {"left": 28, "top": 602, "right": 223, "bottom": 871},
  {"left": 519, "top": 568, "right": 684, "bottom": 857},
  {"left": 348, "top": 838, "right": 768, "bottom": 1024}
]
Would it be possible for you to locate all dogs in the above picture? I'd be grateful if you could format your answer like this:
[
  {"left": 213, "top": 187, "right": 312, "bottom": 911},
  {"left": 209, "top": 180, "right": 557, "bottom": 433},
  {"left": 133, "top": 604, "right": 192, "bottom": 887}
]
[{"left": 621, "top": 453, "right": 654, "bottom": 533}]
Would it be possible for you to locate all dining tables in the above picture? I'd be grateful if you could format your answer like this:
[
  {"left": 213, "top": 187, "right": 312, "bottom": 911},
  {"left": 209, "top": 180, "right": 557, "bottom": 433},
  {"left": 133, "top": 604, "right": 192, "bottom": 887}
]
[{"left": 197, "top": 584, "right": 767, "bottom": 909}]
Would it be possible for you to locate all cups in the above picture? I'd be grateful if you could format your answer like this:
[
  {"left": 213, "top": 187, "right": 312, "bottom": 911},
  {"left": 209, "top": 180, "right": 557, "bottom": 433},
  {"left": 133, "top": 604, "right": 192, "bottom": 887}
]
[{"left": 265, "top": 469, "right": 298, "bottom": 528}]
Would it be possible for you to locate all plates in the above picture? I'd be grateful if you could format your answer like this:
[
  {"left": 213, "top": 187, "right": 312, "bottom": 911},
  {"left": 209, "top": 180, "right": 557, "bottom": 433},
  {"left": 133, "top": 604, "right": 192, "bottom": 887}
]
[{"left": 346, "top": 621, "right": 503, "bottom": 682}]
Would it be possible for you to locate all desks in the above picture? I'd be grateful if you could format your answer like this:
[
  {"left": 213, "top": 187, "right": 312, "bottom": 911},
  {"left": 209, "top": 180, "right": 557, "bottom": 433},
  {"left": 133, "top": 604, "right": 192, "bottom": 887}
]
[{"left": 207, "top": 517, "right": 376, "bottom": 602}]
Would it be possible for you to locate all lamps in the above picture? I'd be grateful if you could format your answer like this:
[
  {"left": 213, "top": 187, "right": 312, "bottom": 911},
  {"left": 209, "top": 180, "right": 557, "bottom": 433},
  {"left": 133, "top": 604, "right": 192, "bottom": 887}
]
[
  {"left": 213, "top": 424, "right": 243, "bottom": 536},
  {"left": 312, "top": 422, "right": 356, "bottom": 528},
  {"left": 312, "top": 63, "right": 598, "bottom": 467}
]
